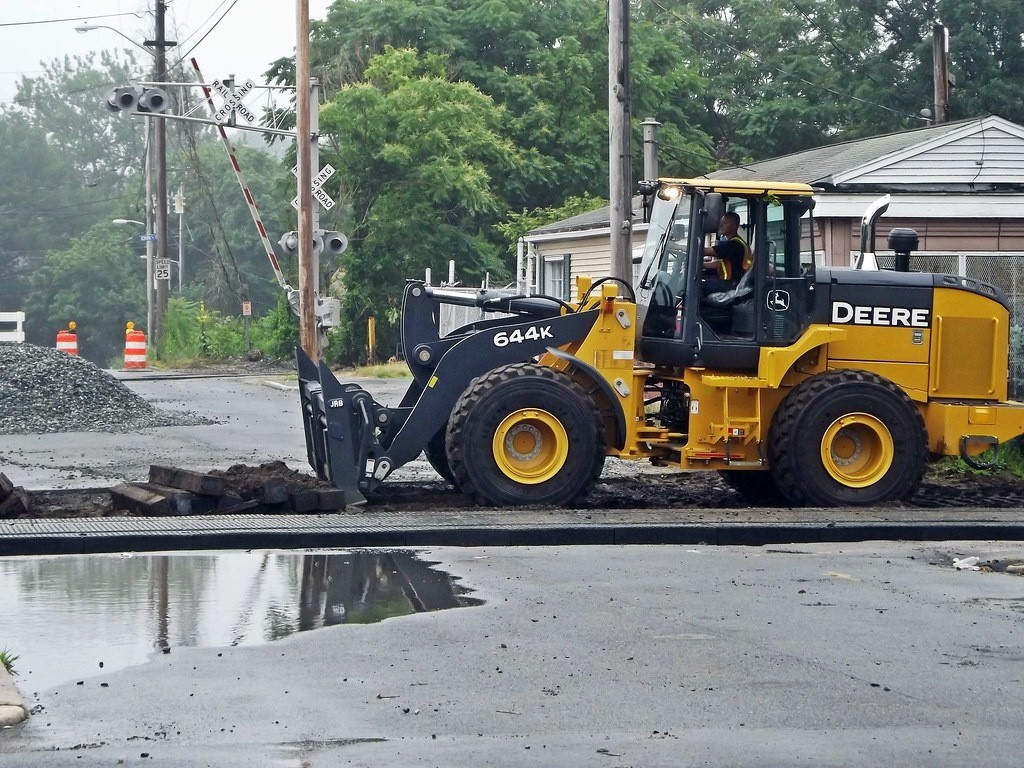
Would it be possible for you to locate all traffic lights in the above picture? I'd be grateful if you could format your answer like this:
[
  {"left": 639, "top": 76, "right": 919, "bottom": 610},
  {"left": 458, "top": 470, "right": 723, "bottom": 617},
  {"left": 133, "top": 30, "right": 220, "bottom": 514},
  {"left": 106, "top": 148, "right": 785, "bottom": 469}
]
[
  {"left": 134, "top": 86, "right": 168, "bottom": 113},
  {"left": 104, "top": 85, "right": 135, "bottom": 112},
  {"left": 312, "top": 232, "right": 324, "bottom": 257},
  {"left": 324, "top": 229, "right": 348, "bottom": 257}
]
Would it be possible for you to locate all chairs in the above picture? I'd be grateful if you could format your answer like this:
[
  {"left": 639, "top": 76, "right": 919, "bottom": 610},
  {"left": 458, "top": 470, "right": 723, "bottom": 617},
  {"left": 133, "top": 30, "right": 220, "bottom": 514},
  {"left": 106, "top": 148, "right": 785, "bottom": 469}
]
[{"left": 698, "top": 224, "right": 757, "bottom": 335}]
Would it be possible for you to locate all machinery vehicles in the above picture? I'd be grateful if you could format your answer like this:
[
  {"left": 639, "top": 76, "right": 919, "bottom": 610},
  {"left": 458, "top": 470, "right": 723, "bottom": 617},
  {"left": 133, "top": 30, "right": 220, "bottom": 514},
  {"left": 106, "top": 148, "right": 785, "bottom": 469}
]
[{"left": 295, "top": 176, "right": 1024, "bottom": 517}]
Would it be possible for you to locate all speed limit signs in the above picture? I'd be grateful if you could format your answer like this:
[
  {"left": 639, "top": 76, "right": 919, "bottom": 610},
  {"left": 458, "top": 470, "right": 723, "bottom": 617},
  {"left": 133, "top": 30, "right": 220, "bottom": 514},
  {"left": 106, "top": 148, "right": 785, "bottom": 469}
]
[{"left": 154, "top": 258, "right": 171, "bottom": 281}]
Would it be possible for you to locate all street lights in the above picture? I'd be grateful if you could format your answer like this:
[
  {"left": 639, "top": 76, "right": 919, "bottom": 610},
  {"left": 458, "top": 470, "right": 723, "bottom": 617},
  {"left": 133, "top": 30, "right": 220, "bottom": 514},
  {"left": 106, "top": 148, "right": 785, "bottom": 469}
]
[{"left": 72, "top": 0, "right": 180, "bottom": 367}]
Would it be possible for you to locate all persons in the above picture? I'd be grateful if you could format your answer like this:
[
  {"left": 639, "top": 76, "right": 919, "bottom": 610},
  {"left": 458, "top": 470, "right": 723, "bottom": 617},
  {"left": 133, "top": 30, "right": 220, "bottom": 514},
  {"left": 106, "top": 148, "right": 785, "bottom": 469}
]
[{"left": 668, "top": 211, "right": 753, "bottom": 309}]
